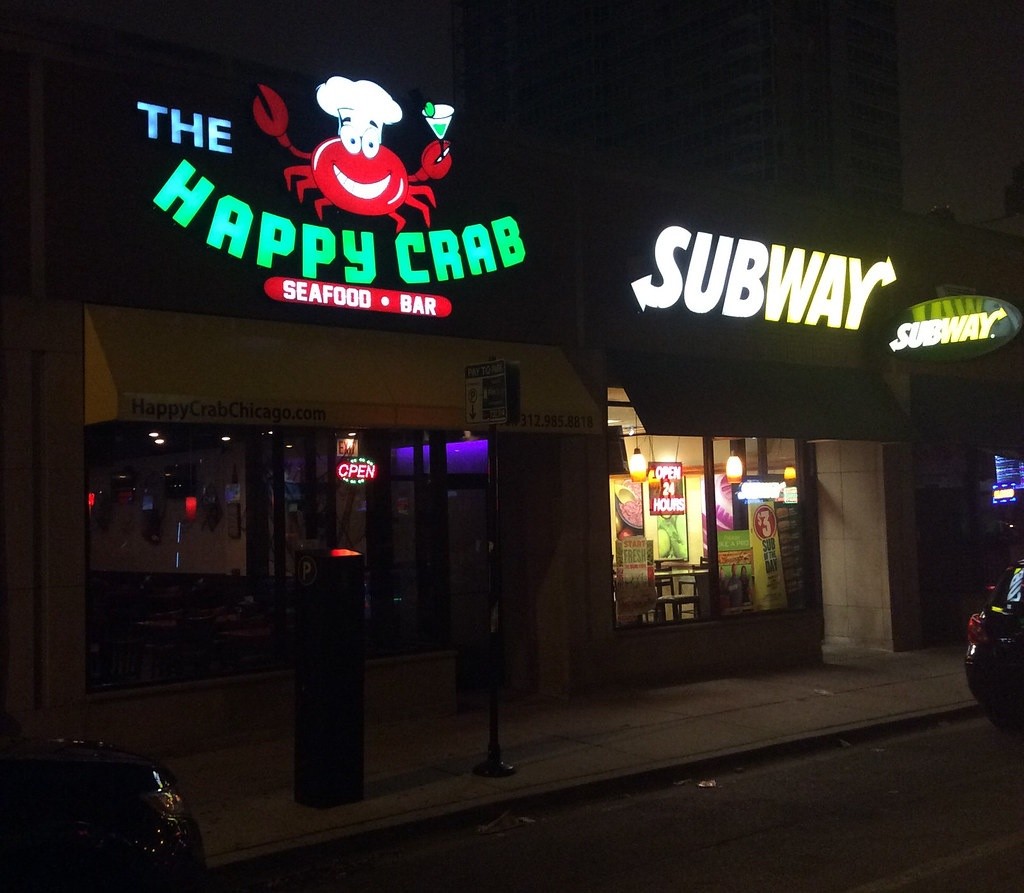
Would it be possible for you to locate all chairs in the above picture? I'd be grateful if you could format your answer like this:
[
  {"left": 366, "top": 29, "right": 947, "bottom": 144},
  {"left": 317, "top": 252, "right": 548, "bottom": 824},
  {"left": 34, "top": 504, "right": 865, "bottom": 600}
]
[
  {"left": 172, "top": 615, "right": 214, "bottom": 681},
  {"left": 645, "top": 556, "right": 709, "bottom": 623},
  {"left": 93, "top": 626, "right": 151, "bottom": 691}
]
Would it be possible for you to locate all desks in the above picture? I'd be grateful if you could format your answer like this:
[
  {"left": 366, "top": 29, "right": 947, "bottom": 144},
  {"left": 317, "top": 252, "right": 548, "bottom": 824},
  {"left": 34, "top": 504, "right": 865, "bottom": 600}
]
[
  {"left": 653, "top": 569, "right": 709, "bottom": 612},
  {"left": 145, "top": 620, "right": 278, "bottom": 651}
]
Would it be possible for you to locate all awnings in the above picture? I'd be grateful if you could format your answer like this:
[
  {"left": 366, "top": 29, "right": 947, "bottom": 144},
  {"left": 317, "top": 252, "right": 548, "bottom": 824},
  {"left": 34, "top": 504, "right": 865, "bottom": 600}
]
[
  {"left": 81, "top": 306, "right": 602, "bottom": 435},
  {"left": 610, "top": 350, "right": 920, "bottom": 441}
]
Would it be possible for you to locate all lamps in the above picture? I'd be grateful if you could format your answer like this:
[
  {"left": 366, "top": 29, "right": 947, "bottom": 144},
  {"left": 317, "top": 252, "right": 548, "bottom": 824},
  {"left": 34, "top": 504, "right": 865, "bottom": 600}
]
[
  {"left": 783, "top": 464, "right": 796, "bottom": 478},
  {"left": 726, "top": 455, "right": 742, "bottom": 483},
  {"left": 629, "top": 416, "right": 647, "bottom": 482}
]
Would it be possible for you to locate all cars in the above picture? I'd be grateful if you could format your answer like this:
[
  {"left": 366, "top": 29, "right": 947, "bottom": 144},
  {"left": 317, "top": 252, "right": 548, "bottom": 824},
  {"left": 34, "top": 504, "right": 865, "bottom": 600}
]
[
  {"left": 1, "top": 711, "right": 211, "bottom": 893},
  {"left": 965, "top": 553, "right": 1024, "bottom": 733}
]
